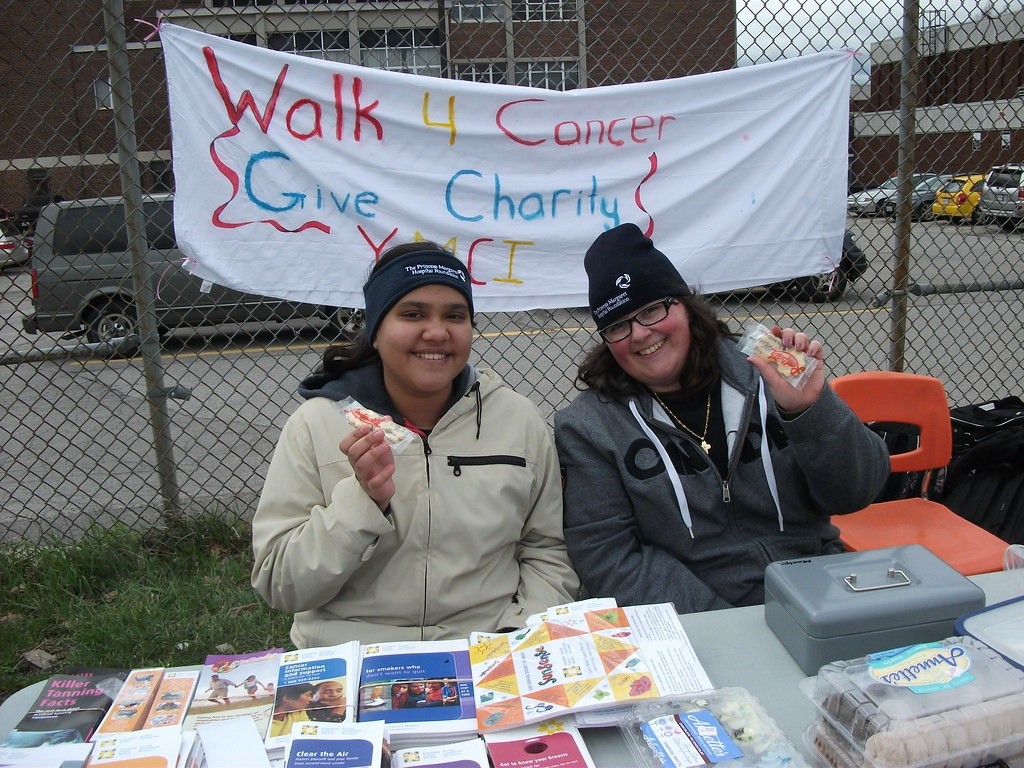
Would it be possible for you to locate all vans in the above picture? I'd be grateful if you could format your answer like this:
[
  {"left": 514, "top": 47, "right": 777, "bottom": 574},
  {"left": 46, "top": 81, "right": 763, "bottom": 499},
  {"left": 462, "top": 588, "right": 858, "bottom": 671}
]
[{"left": 21, "top": 193, "right": 367, "bottom": 360}]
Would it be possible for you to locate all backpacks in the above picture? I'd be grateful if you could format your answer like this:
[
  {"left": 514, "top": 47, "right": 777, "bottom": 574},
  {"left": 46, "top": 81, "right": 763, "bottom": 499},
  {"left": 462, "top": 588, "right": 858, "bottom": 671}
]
[{"left": 857, "top": 395, "right": 1024, "bottom": 553}]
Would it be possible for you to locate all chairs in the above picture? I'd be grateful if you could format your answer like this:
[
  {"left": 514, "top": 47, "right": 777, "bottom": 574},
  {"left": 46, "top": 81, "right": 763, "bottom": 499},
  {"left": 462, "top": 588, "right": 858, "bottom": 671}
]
[{"left": 829, "top": 371, "right": 1017, "bottom": 575}]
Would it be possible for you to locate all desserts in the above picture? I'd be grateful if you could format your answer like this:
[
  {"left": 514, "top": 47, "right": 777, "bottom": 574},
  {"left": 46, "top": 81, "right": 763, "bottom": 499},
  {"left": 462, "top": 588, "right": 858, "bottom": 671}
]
[{"left": 819, "top": 670, "right": 1024, "bottom": 768}]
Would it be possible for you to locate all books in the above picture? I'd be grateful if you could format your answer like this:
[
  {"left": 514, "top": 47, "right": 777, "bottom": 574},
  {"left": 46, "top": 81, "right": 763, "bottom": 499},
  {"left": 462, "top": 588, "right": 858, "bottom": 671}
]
[{"left": 0, "top": 600, "right": 713, "bottom": 768}]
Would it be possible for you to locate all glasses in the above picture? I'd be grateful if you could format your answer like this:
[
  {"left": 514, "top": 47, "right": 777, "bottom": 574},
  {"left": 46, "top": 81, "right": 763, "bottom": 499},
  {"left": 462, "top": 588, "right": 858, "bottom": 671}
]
[{"left": 598, "top": 295, "right": 676, "bottom": 344}]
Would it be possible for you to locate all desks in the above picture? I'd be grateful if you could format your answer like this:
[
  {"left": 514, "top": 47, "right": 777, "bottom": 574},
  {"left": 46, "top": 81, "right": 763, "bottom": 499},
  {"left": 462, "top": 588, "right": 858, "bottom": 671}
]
[{"left": 0, "top": 569, "right": 1023, "bottom": 768}]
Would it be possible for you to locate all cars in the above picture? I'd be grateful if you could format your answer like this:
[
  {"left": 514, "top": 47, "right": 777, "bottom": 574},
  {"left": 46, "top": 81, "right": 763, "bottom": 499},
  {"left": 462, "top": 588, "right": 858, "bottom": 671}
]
[
  {"left": 0, "top": 218, "right": 29, "bottom": 267},
  {"left": 933, "top": 175, "right": 995, "bottom": 226},
  {"left": 884, "top": 174, "right": 953, "bottom": 222},
  {"left": 847, "top": 171, "right": 939, "bottom": 217},
  {"left": 757, "top": 224, "right": 868, "bottom": 303}
]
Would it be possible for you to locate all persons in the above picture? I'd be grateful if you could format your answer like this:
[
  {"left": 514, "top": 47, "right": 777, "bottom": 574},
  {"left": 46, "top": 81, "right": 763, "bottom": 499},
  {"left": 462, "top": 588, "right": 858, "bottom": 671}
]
[
  {"left": 391, "top": 680, "right": 411, "bottom": 709},
  {"left": 205, "top": 674, "right": 238, "bottom": 705},
  {"left": 266, "top": 683, "right": 275, "bottom": 696},
  {"left": 0, "top": 205, "right": 10, "bottom": 237},
  {"left": 402, "top": 678, "right": 425, "bottom": 707},
  {"left": 441, "top": 678, "right": 459, "bottom": 705},
  {"left": 235, "top": 675, "right": 265, "bottom": 700},
  {"left": 270, "top": 683, "right": 314, "bottom": 738},
  {"left": 554, "top": 223, "right": 890, "bottom": 615},
  {"left": 425, "top": 678, "right": 445, "bottom": 705},
  {"left": 251, "top": 241, "right": 581, "bottom": 648},
  {"left": 306, "top": 681, "right": 346, "bottom": 723}
]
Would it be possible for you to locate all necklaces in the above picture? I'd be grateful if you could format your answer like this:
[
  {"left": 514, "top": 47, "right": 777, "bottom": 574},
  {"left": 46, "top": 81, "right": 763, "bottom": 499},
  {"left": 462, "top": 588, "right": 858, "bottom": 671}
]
[{"left": 653, "top": 388, "right": 711, "bottom": 454}]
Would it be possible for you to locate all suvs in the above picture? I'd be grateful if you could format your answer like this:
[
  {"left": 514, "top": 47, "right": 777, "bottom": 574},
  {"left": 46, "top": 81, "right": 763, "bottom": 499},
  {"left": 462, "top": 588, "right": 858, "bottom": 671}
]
[
  {"left": 980, "top": 165, "right": 1024, "bottom": 231},
  {"left": 0, "top": 203, "right": 41, "bottom": 248}
]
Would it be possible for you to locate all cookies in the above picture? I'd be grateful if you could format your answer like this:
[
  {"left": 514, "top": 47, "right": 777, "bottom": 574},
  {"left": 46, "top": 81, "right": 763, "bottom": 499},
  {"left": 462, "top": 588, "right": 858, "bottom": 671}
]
[
  {"left": 775, "top": 348, "right": 807, "bottom": 377},
  {"left": 753, "top": 334, "right": 781, "bottom": 363},
  {"left": 348, "top": 408, "right": 406, "bottom": 444}
]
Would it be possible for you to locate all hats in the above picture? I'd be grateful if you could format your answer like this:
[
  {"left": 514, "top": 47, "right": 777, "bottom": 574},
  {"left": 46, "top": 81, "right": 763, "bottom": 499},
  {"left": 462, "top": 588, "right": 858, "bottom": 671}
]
[{"left": 583, "top": 223, "right": 692, "bottom": 330}]
